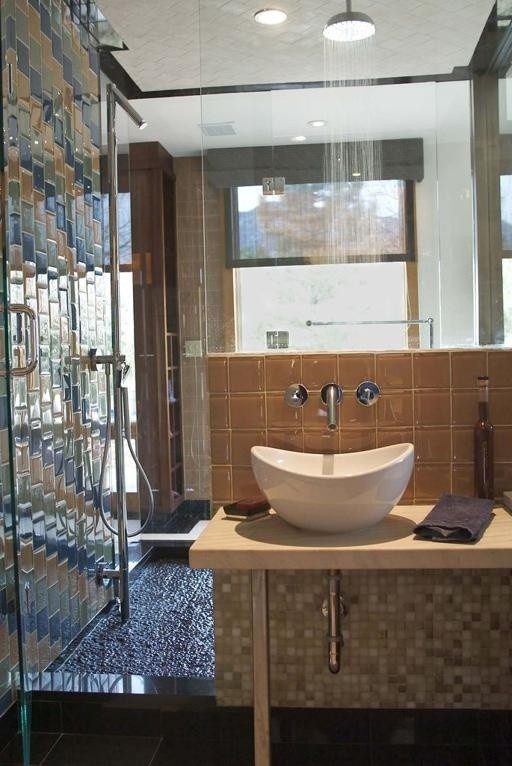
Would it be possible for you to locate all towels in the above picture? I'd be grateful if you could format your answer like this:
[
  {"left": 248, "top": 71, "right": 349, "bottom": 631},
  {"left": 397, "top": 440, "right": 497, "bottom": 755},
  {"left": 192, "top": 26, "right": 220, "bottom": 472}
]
[{"left": 413, "top": 492, "right": 495, "bottom": 541}]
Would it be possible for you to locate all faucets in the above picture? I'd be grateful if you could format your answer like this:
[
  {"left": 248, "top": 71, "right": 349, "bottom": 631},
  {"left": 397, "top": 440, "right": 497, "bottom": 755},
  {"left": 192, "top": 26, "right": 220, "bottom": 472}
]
[{"left": 321, "top": 383, "right": 344, "bottom": 432}]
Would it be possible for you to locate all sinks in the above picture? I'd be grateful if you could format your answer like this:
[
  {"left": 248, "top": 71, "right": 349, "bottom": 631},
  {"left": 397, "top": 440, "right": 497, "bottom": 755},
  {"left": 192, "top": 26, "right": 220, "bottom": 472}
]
[{"left": 249, "top": 442, "right": 417, "bottom": 534}]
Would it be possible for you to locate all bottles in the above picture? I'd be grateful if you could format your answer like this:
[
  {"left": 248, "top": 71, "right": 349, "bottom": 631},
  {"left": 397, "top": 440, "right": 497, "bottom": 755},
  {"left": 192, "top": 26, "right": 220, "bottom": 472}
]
[{"left": 474, "top": 373, "right": 495, "bottom": 502}]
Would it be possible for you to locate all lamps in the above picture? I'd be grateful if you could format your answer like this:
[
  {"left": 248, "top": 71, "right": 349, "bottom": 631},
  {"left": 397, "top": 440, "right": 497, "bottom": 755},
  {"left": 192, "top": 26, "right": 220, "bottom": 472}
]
[{"left": 254, "top": 7, "right": 286, "bottom": 26}]
